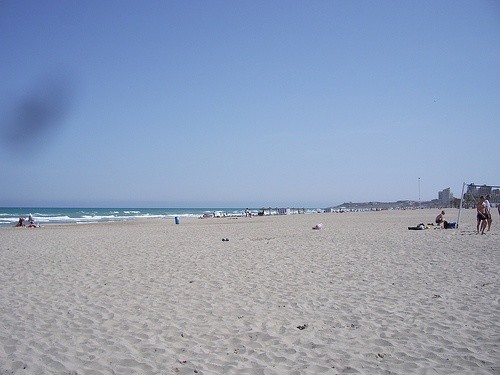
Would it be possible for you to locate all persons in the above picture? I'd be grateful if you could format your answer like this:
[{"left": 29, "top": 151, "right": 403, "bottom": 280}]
[
  {"left": 476, "top": 194, "right": 493, "bottom": 235},
  {"left": 318, "top": 208, "right": 349, "bottom": 213},
  {"left": 436, "top": 210, "right": 446, "bottom": 226},
  {"left": 278, "top": 208, "right": 306, "bottom": 215},
  {"left": 199, "top": 210, "right": 230, "bottom": 219},
  {"left": 490, "top": 203, "right": 500, "bottom": 216},
  {"left": 16, "top": 212, "right": 40, "bottom": 228},
  {"left": 245, "top": 208, "right": 252, "bottom": 217},
  {"left": 351, "top": 207, "right": 382, "bottom": 212},
  {"left": 259, "top": 207, "right": 272, "bottom": 215}
]
